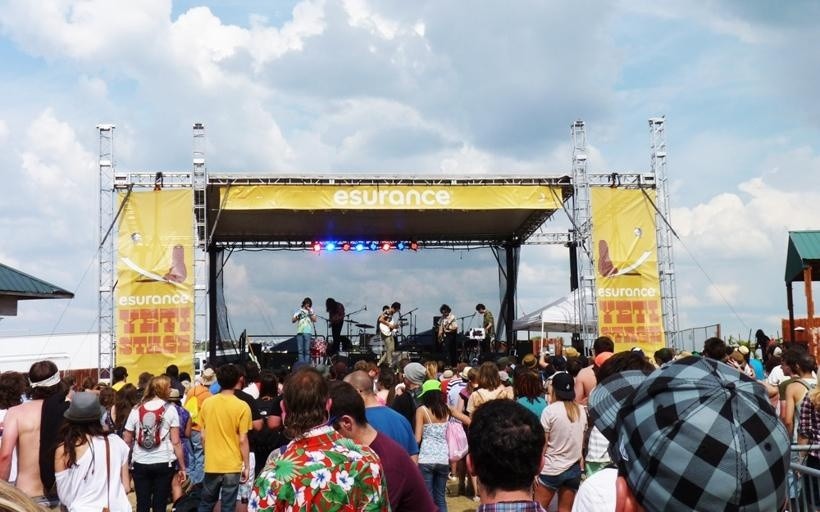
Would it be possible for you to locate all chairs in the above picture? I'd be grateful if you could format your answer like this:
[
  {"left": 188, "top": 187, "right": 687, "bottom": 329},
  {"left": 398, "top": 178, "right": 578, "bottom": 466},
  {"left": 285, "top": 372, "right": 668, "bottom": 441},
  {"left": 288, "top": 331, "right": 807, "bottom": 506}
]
[{"left": 447, "top": 472, "right": 480, "bottom": 503}]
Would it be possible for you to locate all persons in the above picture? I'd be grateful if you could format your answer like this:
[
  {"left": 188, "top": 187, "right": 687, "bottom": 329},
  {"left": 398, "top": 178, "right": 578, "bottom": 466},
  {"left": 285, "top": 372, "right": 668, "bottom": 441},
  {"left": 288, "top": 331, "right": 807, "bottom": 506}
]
[{"left": 0, "top": 294, "right": 819, "bottom": 512}]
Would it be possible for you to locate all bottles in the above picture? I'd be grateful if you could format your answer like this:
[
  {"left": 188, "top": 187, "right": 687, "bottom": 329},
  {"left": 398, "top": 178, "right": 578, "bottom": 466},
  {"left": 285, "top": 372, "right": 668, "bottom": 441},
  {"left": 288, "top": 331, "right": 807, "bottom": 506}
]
[
  {"left": 552, "top": 372, "right": 576, "bottom": 401},
  {"left": 167, "top": 388, "right": 181, "bottom": 402},
  {"left": 402, "top": 362, "right": 428, "bottom": 384},
  {"left": 440, "top": 366, "right": 473, "bottom": 382},
  {"left": 496, "top": 354, "right": 538, "bottom": 370},
  {"left": 198, "top": 367, "right": 218, "bottom": 386},
  {"left": 727, "top": 339, "right": 783, "bottom": 369},
  {"left": 562, "top": 347, "right": 579, "bottom": 359},
  {"left": 417, "top": 379, "right": 441, "bottom": 398},
  {"left": 586, "top": 355, "right": 792, "bottom": 512},
  {"left": 63, "top": 391, "right": 106, "bottom": 421}
]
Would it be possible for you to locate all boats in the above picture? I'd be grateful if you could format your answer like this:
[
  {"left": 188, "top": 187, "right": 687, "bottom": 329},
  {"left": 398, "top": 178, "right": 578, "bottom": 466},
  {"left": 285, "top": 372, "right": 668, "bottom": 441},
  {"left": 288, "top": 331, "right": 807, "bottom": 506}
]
[
  {"left": 517, "top": 340, "right": 533, "bottom": 359},
  {"left": 572, "top": 339, "right": 584, "bottom": 356},
  {"left": 248, "top": 344, "right": 262, "bottom": 364}
]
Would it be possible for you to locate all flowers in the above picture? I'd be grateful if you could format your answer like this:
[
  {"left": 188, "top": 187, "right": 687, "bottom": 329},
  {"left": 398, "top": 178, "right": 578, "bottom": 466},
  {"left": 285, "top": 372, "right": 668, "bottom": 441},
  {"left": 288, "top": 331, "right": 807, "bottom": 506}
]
[{"left": 364, "top": 305, "right": 367, "bottom": 311}]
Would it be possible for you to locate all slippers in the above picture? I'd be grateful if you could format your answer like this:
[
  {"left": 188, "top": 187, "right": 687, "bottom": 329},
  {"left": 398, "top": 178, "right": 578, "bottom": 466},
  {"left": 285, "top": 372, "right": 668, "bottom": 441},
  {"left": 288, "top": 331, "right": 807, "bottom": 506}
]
[
  {"left": 134, "top": 401, "right": 171, "bottom": 450},
  {"left": 184, "top": 385, "right": 208, "bottom": 423}
]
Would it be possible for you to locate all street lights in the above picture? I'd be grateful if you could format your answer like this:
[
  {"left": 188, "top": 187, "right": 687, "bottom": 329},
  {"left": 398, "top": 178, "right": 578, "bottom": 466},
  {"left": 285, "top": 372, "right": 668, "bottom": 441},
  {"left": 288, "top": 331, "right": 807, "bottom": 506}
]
[{"left": 445, "top": 422, "right": 469, "bottom": 462}]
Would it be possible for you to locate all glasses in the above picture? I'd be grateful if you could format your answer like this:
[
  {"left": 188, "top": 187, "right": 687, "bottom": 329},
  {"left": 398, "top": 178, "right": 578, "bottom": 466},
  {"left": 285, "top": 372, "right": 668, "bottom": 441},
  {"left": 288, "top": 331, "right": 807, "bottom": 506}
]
[{"left": 312, "top": 237, "right": 420, "bottom": 251}]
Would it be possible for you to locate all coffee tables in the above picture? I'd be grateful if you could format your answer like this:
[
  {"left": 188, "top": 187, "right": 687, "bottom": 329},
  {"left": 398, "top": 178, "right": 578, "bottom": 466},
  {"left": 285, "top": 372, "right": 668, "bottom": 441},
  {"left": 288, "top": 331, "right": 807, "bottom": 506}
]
[
  {"left": 345, "top": 318, "right": 358, "bottom": 323},
  {"left": 355, "top": 324, "right": 375, "bottom": 328}
]
